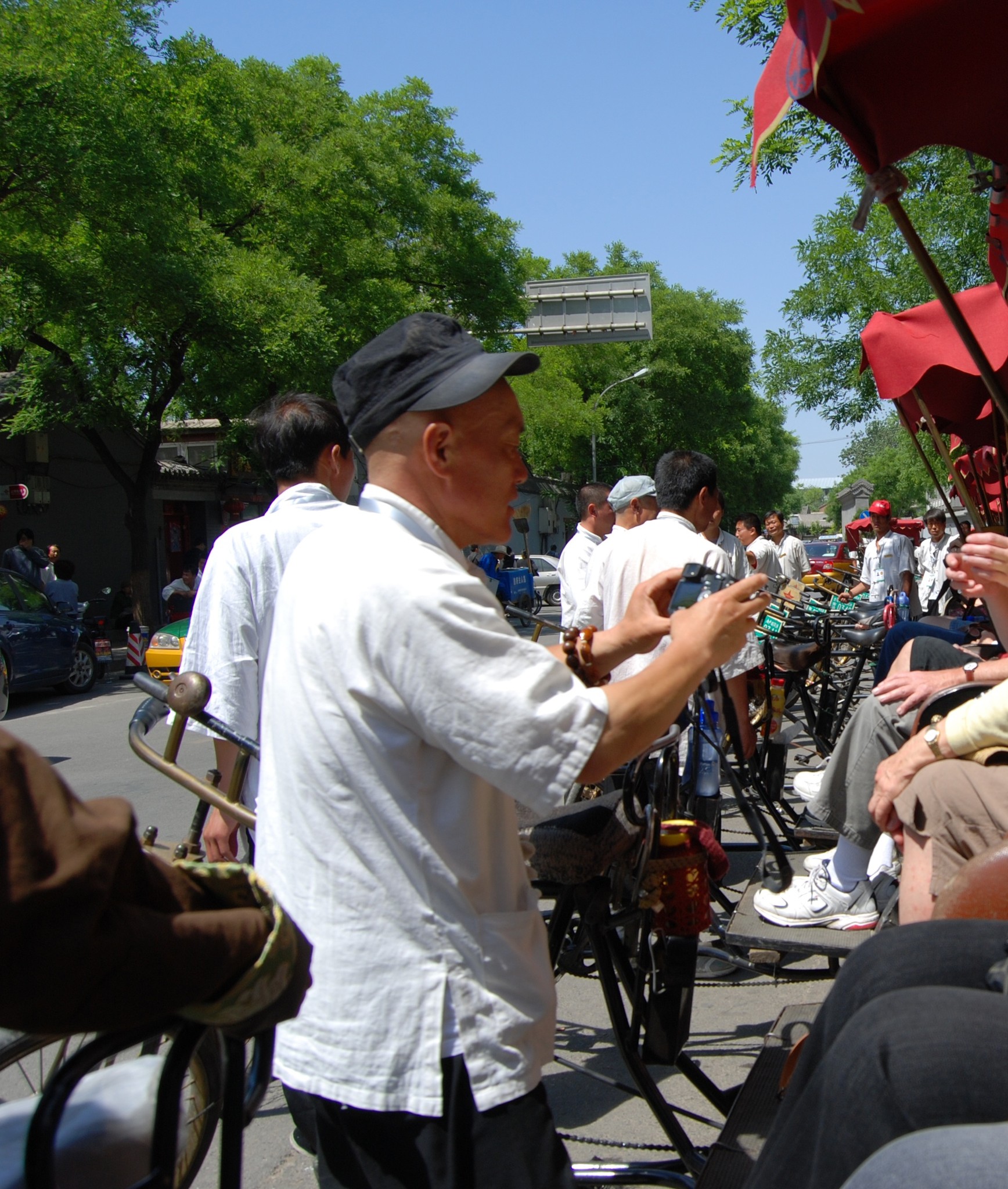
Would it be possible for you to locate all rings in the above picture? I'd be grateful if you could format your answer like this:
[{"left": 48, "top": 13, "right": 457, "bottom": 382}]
[{"left": 958, "top": 589, "right": 964, "bottom": 595}]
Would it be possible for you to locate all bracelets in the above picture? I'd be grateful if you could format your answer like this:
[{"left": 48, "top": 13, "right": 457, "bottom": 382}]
[
  {"left": 563, "top": 628, "right": 594, "bottom": 688},
  {"left": 578, "top": 626, "right": 613, "bottom": 686}
]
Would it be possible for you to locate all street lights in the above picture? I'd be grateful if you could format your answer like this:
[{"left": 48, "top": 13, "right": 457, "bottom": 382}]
[{"left": 592, "top": 367, "right": 651, "bottom": 483}]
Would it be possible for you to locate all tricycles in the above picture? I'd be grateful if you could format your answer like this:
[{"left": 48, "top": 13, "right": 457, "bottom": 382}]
[
  {"left": 0, "top": 0, "right": 1008, "bottom": 1187},
  {"left": 497, "top": 566, "right": 542, "bottom": 626}
]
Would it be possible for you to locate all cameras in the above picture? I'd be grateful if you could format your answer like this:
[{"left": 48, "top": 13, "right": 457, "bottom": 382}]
[{"left": 667, "top": 561, "right": 750, "bottom": 612}]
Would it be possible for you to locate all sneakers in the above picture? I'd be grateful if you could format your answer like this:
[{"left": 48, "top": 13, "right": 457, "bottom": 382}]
[
  {"left": 695, "top": 956, "right": 737, "bottom": 977},
  {"left": 793, "top": 756, "right": 831, "bottom": 801},
  {"left": 803, "top": 849, "right": 902, "bottom": 883},
  {"left": 753, "top": 859, "right": 879, "bottom": 930}
]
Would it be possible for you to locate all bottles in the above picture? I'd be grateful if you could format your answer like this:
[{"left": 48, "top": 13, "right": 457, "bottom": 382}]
[
  {"left": 762, "top": 678, "right": 786, "bottom": 739},
  {"left": 681, "top": 699, "right": 722, "bottom": 797},
  {"left": 771, "top": 720, "right": 805, "bottom": 744},
  {"left": 895, "top": 591, "right": 910, "bottom": 622},
  {"left": 650, "top": 819, "right": 711, "bottom": 938}
]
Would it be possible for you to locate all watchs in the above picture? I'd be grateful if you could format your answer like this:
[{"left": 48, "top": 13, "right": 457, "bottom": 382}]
[
  {"left": 963, "top": 662, "right": 979, "bottom": 681},
  {"left": 924, "top": 722, "right": 945, "bottom": 761}
]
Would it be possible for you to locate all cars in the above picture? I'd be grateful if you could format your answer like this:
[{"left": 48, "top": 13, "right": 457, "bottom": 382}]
[
  {"left": 144, "top": 617, "right": 191, "bottom": 684},
  {"left": 0, "top": 568, "right": 98, "bottom": 694},
  {"left": 801, "top": 542, "right": 858, "bottom": 592},
  {"left": 512, "top": 555, "right": 562, "bottom": 606}
]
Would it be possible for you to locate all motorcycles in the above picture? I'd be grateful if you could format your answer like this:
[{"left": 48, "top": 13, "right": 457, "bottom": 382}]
[{"left": 68, "top": 587, "right": 113, "bottom": 679}]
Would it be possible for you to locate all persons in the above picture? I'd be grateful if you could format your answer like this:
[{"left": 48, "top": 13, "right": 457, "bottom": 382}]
[
  {"left": 255, "top": 314, "right": 773, "bottom": 1189},
  {"left": 0, "top": 394, "right": 536, "bottom": 1034},
  {"left": 558, "top": 447, "right": 1008, "bottom": 1189}
]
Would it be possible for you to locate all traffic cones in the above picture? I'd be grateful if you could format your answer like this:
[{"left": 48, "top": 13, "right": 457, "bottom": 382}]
[{"left": 120, "top": 621, "right": 147, "bottom": 680}]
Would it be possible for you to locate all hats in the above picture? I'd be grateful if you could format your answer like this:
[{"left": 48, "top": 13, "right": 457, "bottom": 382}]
[
  {"left": 607, "top": 475, "right": 657, "bottom": 512},
  {"left": 868, "top": 499, "right": 891, "bottom": 517},
  {"left": 490, "top": 545, "right": 510, "bottom": 556},
  {"left": 333, "top": 312, "right": 540, "bottom": 454}
]
